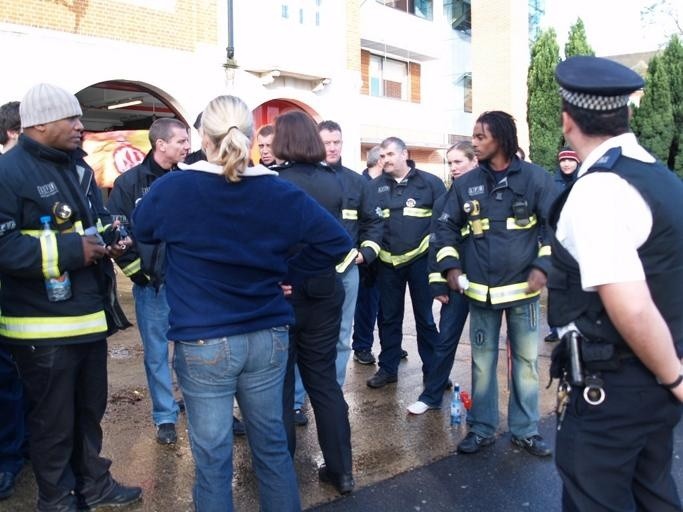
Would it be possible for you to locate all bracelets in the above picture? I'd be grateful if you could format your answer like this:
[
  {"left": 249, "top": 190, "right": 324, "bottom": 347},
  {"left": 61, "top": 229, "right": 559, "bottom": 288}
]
[{"left": 657, "top": 375, "right": 682, "bottom": 390}]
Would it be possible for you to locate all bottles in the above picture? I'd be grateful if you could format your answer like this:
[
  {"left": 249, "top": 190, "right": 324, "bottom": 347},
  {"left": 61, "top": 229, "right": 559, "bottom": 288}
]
[
  {"left": 36, "top": 215, "right": 71, "bottom": 305},
  {"left": 449, "top": 383, "right": 462, "bottom": 427}
]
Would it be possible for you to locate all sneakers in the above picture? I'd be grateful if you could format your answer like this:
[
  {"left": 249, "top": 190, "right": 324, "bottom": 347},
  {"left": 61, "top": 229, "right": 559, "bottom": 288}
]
[
  {"left": 405, "top": 399, "right": 441, "bottom": 416},
  {"left": 231, "top": 413, "right": 247, "bottom": 437},
  {"left": 294, "top": 408, "right": 309, "bottom": 426},
  {"left": 37, "top": 492, "right": 78, "bottom": 512},
  {"left": 156, "top": 421, "right": 177, "bottom": 445},
  {"left": 0, "top": 468, "right": 19, "bottom": 501},
  {"left": 365, "top": 368, "right": 398, "bottom": 388},
  {"left": 457, "top": 431, "right": 497, "bottom": 455},
  {"left": 423, "top": 370, "right": 454, "bottom": 390},
  {"left": 78, "top": 477, "right": 143, "bottom": 511},
  {"left": 318, "top": 462, "right": 358, "bottom": 494},
  {"left": 398, "top": 348, "right": 409, "bottom": 358},
  {"left": 352, "top": 348, "right": 379, "bottom": 366},
  {"left": 510, "top": 433, "right": 552, "bottom": 457}
]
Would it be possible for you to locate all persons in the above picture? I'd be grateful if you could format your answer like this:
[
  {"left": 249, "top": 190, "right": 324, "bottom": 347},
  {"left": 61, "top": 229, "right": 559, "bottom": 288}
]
[
  {"left": 408, "top": 111, "right": 553, "bottom": 456},
  {"left": 557, "top": 142, "right": 583, "bottom": 180},
  {"left": 544, "top": 52, "right": 681, "bottom": 512},
  {"left": 0, "top": 82, "right": 453, "bottom": 511}
]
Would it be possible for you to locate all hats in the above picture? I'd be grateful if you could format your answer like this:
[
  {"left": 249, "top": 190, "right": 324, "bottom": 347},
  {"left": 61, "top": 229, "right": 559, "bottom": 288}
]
[
  {"left": 19, "top": 80, "right": 86, "bottom": 130},
  {"left": 554, "top": 57, "right": 647, "bottom": 113},
  {"left": 558, "top": 145, "right": 584, "bottom": 165}
]
